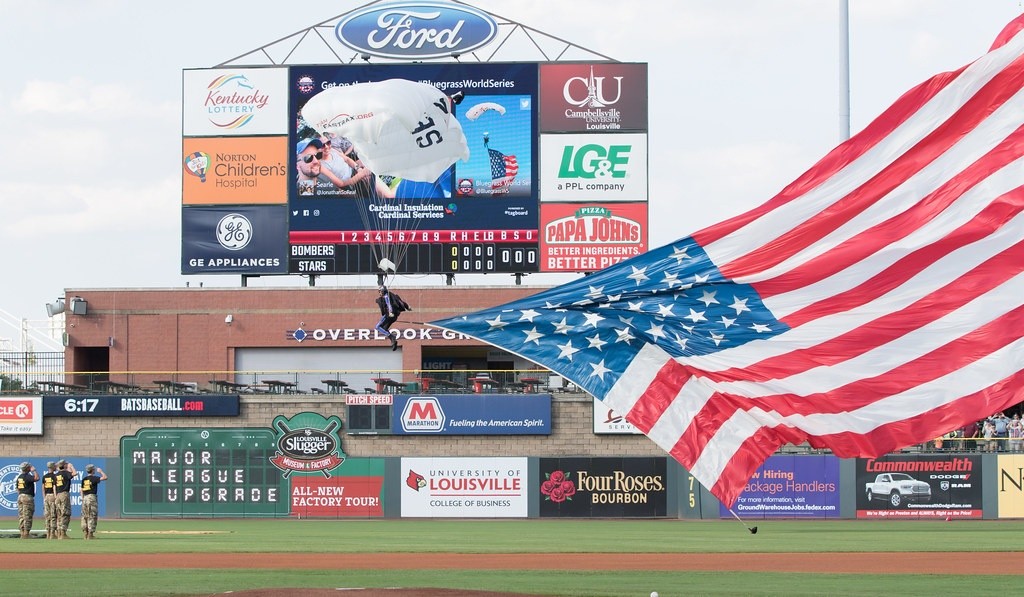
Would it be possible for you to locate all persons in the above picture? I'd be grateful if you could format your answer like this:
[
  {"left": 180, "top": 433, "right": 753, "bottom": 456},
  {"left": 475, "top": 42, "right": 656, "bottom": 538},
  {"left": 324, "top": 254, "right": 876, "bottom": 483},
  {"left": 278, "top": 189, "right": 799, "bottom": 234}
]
[
  {"left": 15, "top": 462, "right": 40, "bottom": 538},
  {"left": 53, "top": 459, "right": 77, "bottom": 539},
  {"left": 80, "top": 463, "right": 108, "bottom": 539},
  {"left": 374, "top": 284, "right": 412, "bottom": 350},
  {"left": 922, "top": 412, "right": 1024, "bottom": 454},
  {"left": 42, "top": 462, "right": 56, "bottom": 539},
  {"left": 297, "top": 135, "right": 399, "bottom": 199}
]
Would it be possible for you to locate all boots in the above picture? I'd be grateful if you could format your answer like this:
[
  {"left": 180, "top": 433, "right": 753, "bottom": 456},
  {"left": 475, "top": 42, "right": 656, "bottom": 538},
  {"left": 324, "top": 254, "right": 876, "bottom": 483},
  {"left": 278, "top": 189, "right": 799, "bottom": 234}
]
[
  {"left": 20, "top": 530, "right": 24, "bottom": 539},
  {"left": 87, "top": 532, "right": 97, "bottom": 540},
  {"left": 24, "top": 530, "right": 33, "bottom": 539},
  {"left": 392, "top": 341, "right": 397, "bottom": 351},
  {"left": 83, "top": 531, "right": 88, "bottom": 540},
  {"left": 47, "top": 529, "right": 57, "bottom": 539},
  {"left": 388, "top": 334, "right": 396, "bottom": 345},
  {"left": 58, "top": 530, "right": 70, "bottom": 540}
]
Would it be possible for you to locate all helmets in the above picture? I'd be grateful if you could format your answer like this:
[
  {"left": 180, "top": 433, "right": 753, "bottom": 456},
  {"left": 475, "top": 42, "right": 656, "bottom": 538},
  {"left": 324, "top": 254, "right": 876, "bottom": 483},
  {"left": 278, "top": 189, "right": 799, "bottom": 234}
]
[{"left": 377, "top": 285, "right": 387, "bottom": 296}]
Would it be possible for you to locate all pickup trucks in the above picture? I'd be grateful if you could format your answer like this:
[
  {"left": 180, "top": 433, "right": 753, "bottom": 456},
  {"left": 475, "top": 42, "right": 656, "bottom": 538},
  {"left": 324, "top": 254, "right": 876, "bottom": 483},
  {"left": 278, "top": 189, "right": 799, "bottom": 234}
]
[{"left": 864, "top": 473, "right": 931, "bottom": 510}]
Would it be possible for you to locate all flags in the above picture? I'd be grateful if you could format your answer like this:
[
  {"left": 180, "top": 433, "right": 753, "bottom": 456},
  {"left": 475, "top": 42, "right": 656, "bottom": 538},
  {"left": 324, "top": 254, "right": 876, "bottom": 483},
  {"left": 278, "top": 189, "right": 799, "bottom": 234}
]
[
  {"left": 423, "top": 7, "right": 1024, "bottom": 510},
  {"left": 488, "top": 148, "right": 519, "bottom": 196}
]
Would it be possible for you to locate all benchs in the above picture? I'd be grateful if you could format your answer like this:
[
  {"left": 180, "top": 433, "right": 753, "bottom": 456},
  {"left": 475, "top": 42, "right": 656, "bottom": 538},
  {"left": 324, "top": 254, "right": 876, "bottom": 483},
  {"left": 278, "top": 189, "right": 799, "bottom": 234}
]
[
  {"left": 297, "top": 390, "right": 307, "bottom": 395},
  {"left": 512, "top": 383, "right": 525, "bottom": 393},
  {"left": 117, "top": 388, "right": 132, "bottom": 395},
  {"left": 251, "top": 389, "right": 264, "bottom": 394},
  {"left": 310, "top": 388, "right": 327, "bottom": 394},
  {"left": 287, "top": 388, "right": 295, "bottom": 394},
  {"left": 232, "top": 389, "right": 247, "bottom": 394},
  {"left": 393, "top": 383, "right": 407, "bottom": 394},
  {"left": 364, "top": 388, "right": 376, "bottom": 394},
  {"left": 176, "top": 389, "right": 194, "bottom": 395},
  {"left": 199, "top": 389, "right": 211, "bottom": 394},
  {"left": 559, "top": 386, "right": 571, "bottom": 394},
  {"left": 141, "top": 388, "right": 151, "bottom": 394},
  {"left": 85, "top": 389, "right": 104, "bottom": 396},
  {"left": 542, "top": 386, "right": 554, "bottom": 394},
  {"left": 344, "top": 388, "right": 356, "bottom": 395}
]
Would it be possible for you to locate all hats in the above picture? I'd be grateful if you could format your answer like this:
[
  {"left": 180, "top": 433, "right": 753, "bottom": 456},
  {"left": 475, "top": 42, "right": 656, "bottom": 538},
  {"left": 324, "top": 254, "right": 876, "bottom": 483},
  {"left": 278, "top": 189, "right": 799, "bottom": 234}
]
[
  {"left": 20, "top": 462, "right": 31, "bottom": 470},
  {"left": 58, "top": 459, "right": 68, "bottom": 467},
  {"left": 47, "top": 462, "right": 54, "bottom": 467},
  {"left": 86, "top": 464, "right": 96, "bottom": 471},
  {"left": 297, "top": 138, "right": 323, "bottom": 153}
]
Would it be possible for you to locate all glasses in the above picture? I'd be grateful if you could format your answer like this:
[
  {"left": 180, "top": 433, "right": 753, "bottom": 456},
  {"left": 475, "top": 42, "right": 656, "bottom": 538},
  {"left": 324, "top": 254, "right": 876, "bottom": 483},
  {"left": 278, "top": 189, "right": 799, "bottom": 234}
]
[
  {"left": 321, "top": 140, "right": 331, "bottom": 149},
  {"left": 298, "top": 153, "right": 323, "bottom": 163}
]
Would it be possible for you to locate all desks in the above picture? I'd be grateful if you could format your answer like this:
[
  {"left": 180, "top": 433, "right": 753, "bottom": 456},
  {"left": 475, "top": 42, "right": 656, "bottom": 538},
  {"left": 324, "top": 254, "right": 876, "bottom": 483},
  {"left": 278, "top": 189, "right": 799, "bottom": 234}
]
[
  {"left": 371, "top": 378, "right": 398, "bottom": 396},
  {"left": 95, "top": 381, "right": 128, "bottom": 395},
  {"left": 261, "top": 380, "right": 287, "bottom": 396},
  {"left": 468, "top": 378, "right": 500, "bottom": 393},
  {"left": 36, "top": 380, "right": 71, "bottom": 395},
  {"left": 321, "top": 380, "right": 349, "bottom": 394},
  {"left": 153, "top": 380, "right": 182, "bottom": 395},
  {"left": 208, "top": 380, "right": 232, "bottom": 394},
  {"left": 428, "top": 379, "right": 463, "bottom": 393},
  {"left": 521, "top": 378, "right": 544, "bottom": 394}
]
